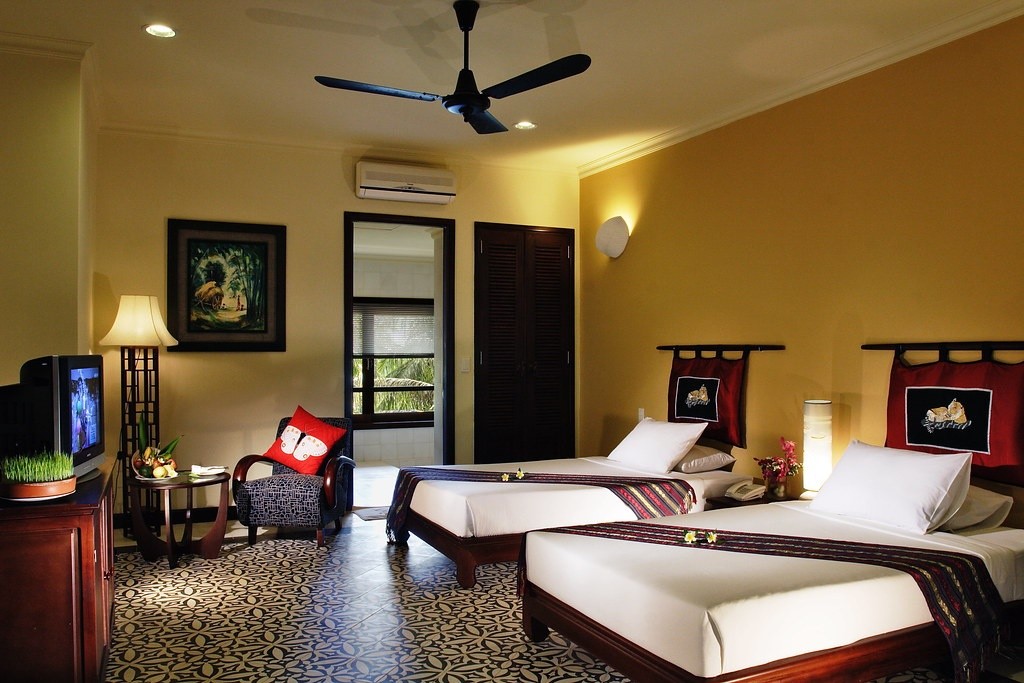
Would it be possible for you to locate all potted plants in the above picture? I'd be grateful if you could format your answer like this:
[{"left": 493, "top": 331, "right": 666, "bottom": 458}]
[{"left": 2, "top": 450, "right": 76, "bottom": 502}]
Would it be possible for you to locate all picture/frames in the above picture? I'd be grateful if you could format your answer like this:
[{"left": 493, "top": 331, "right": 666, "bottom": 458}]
[{"left": 168, "top": 217, "right": 287, "bottom": 353}]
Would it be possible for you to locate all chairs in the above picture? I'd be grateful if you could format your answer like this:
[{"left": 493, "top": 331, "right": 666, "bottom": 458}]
[{"left": 230, "top": 415, "right": 355, "bottom": 548}]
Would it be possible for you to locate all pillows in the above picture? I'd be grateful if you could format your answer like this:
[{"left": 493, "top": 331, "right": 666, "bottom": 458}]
[
  {"left": 678, "top": 443, "right": 735, "bottom": 472},
  {"left": 946, "top": 485, "right": 1015, "bottom": 536},
  {"left": 608, "top": 416, "right": 710, "bottom": 473},
  {"left": 824, "top": 440, "right": 973, "bottom": 540},
  {"left": 263, "top": 405, "right": 346, "bottom": 474}
]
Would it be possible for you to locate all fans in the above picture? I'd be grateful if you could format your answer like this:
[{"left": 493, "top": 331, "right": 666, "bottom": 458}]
[{"left": 314, "top": 1, "right": 591, "bottom": 134}]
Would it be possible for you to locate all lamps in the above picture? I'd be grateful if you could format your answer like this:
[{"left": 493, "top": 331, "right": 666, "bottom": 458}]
[
  {"left": 799, "top": 398, "right": 832, "bottom": 500},
  {"left": 597, "top": 216, "right": 628, "bottom": 257},
  {"left": 101, "top": 295, "right": 179, "bottom": 541}
]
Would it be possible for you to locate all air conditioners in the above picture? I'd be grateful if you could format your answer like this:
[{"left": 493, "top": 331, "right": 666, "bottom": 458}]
[{"left": 353, "top": 159, "right": 455, "bottom": 205}]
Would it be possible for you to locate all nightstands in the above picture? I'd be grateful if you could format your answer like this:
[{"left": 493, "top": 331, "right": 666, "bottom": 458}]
[{"left": 702, "top": 494, "right": 798, "bottom": 515}]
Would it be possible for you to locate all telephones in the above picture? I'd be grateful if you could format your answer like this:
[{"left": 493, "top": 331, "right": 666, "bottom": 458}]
[{"left": 725, "top": 479, "right": 766, "bottom": 501}]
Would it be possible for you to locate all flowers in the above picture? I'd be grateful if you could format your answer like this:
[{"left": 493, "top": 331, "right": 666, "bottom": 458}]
[{"left": 750, "top": 437, "right": 802, "bottom": 490}]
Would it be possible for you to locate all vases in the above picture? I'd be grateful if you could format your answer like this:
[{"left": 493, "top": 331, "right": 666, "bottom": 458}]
[{"left": 774, "top": 479, "right": 792, "bottom": 498}]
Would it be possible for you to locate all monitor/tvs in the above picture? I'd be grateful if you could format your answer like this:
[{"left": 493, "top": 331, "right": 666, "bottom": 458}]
[{"left": 0, "top": 354, "right": 106, "bottom": 483}]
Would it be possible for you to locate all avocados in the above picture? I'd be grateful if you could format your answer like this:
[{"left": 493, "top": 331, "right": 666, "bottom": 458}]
[{"left": 139, "top": 465, "right": 153, "bottom": 478}]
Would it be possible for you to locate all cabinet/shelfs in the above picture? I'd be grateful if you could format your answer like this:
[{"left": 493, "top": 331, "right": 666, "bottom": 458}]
[{"left": 0, "top": 454, "right": 117, "bottom": 683}]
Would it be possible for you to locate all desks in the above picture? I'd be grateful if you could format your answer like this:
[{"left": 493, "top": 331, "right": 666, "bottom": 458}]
[{"left": 123, "top": 472, "right": 233, "bottom": 568}]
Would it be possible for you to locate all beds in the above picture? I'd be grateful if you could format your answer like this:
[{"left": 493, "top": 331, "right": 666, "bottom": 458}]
[
  {"left": 517, "top": 338, "right": 1023, "bottom": 683},
  {"left": 386, "top": 343, "right": 785, "bottom": 588}
]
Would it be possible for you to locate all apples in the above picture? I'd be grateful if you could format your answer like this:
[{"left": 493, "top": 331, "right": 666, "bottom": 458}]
[{"left": 135, "top": 458, "right": 177, "bottom": 478}]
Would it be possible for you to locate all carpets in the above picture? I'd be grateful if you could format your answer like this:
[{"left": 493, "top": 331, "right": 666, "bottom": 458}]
[{"left": 354, "top": 506, "right": 390, "bottom": 521}]
